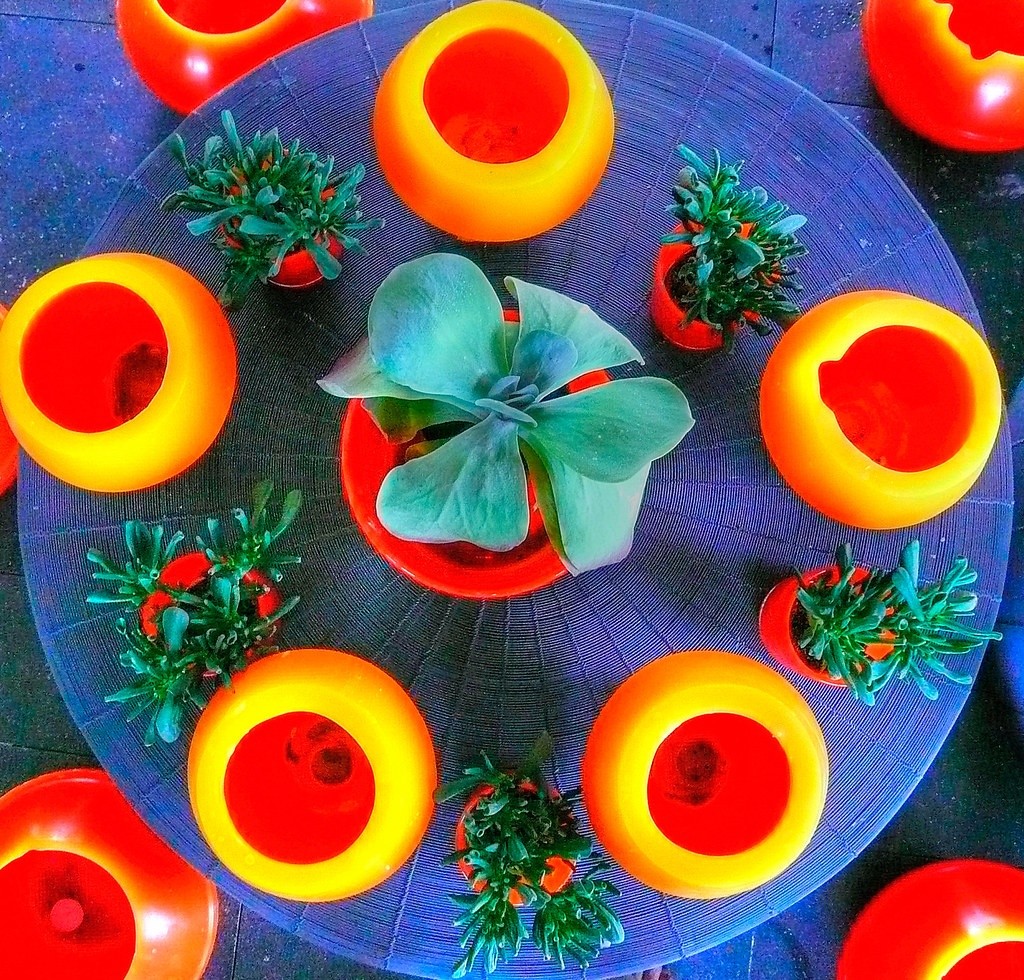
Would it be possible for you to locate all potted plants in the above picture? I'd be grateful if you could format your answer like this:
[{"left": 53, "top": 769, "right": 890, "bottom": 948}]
[
  {"left": 651, "top": 143, "right": 808, "bottom": 353},
  {"left": 432, "top": 731, "right": 624, "bottom": 980},
  {"left": 84, "top": 479, "right": 301, "bottom": 747},
  {"left": 315, "top": 252, "right": 695, "bottom": 598},
  {"left": 760, "top": 537, "right": 1003, "bottom": 708},
  {"left": 160, "top": 109, "right": 386, "bottom": 313}
]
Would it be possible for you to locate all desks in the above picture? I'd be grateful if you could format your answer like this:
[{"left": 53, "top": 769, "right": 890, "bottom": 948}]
[{"left": 17, "top": 0, "right": 1014, "bottom": 980}]
